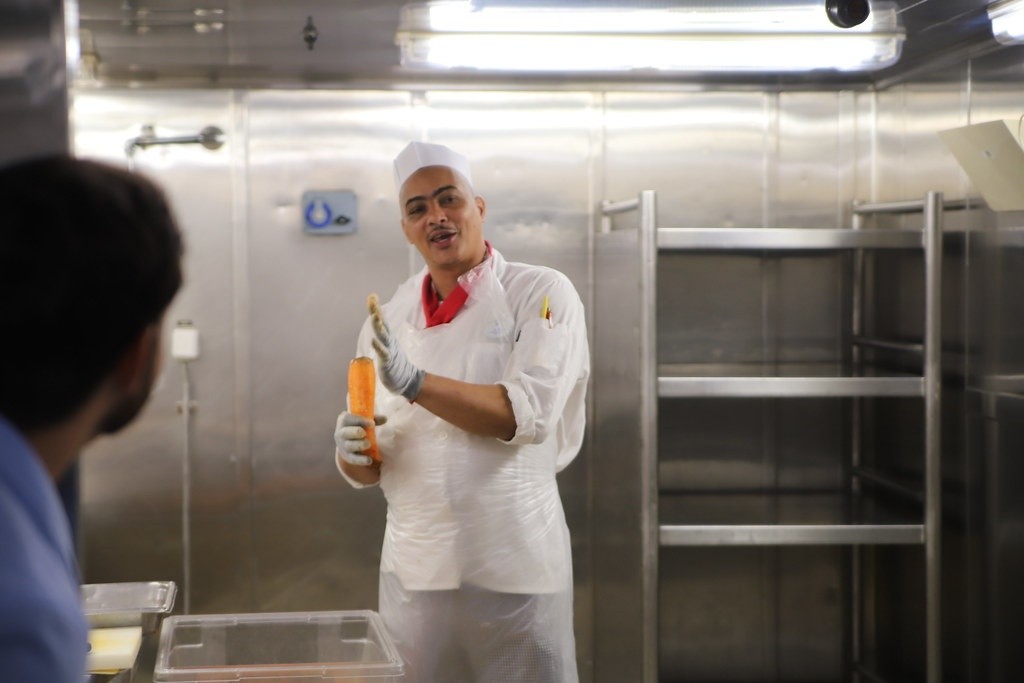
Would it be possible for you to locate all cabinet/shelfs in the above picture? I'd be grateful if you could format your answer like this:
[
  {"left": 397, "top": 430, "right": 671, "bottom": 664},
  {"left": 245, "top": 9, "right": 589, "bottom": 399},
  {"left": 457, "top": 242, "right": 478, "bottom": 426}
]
[{"left": 593, "top": 190, "right": 940, "bottom": 683}]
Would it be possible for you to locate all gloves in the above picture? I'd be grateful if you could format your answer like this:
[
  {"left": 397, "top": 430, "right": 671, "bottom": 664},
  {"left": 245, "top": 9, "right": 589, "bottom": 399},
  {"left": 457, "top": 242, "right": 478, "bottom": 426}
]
[
  {"left": 368, "top": 293, "right": 421, "bottom": 404},
  {"left": 334, "top": 411, "right": 388, "bottom": 467}
]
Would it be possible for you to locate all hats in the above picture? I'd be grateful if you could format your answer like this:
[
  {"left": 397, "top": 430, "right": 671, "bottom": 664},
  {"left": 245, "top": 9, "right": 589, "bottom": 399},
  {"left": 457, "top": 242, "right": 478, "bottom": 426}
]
[{"left": 393, "top": 141, "right": 472, "bottom": 200}]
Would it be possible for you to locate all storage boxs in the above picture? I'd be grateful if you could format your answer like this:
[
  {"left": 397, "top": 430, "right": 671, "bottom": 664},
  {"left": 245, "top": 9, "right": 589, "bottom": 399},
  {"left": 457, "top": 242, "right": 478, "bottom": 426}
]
[{"left": 151, "top": 609, "right": 405, "bottom": 683}]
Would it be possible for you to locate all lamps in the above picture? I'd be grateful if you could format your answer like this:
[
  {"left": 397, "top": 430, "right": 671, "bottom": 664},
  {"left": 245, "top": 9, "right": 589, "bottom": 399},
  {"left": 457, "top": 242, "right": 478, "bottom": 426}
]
[{"left": 394, "top": 0, "right": 1024, "bottom": 77}]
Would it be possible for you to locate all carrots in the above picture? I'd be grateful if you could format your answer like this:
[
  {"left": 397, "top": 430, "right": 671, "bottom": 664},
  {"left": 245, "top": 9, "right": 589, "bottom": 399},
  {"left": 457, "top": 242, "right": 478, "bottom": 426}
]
[{"left": 348, "top": 356, "right": 380, "bottom": 462}]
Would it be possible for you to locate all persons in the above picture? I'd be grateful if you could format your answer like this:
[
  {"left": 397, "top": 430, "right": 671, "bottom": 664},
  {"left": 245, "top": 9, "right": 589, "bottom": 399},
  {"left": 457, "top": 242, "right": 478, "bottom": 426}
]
[
  {"left": 335, "top": 141, "right": 589, "bottom": 683},
  {"left": 0, "top": 150, "right": 185, "bottom": 683}
]
